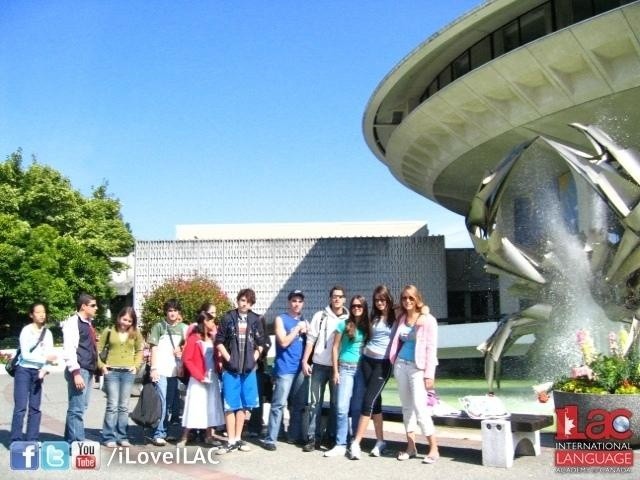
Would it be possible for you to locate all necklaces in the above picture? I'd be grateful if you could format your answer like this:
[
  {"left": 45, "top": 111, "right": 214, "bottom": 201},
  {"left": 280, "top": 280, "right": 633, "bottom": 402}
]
[{"left": 405, "top": 318, "right": 418, "bottom": 328}]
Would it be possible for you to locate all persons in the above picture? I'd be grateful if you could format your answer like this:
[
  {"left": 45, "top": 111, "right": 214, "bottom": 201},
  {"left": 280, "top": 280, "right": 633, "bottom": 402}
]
[
  {"left": 61, "top": 292, "right": 100, "bottom": 456},
  {"left": 6, "top": 302, "right": 58, "bottom": 453},
  {"left": 323, "top": 294, "right": 371, "bottom": 459},
  {"left": 263, "top": 288, "right": 313, "bottom": 452},
  {"left": 93, "top": 286, "right": 272, "bottom": 454},
  {"left": 303, "top": 287, "right": 351, "bottom": 454},
  {"left": 347, "top": 284, "right": 430, "bottom": 460},
  {"left": 386, "top": 284, "right": 443, "bottom": 465}
]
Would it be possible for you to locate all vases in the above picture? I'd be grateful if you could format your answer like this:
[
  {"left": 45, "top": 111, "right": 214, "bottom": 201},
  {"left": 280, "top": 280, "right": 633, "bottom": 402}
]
[{"left": 551, "top": 390, "right": 640, "bottom": 449}]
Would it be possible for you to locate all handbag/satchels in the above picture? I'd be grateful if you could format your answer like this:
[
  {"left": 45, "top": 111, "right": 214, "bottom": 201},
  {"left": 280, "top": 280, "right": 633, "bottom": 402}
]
[
  {"left": 458, "top": 395, "right": 511, "bottom": 421},
  {"left": 93, "top": 324, "right": 112, "bottom": 376},
  {"left": 5, "top": 349, "right": 21, "bottom": 377},
  {"left": 175, "top": 359, "right": 189, "bottom": 378}
]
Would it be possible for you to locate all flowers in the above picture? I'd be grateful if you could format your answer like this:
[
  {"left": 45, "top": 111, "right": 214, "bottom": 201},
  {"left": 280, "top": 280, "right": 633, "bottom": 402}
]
[{"left": 539, "top": 326, "right": 640, "bottom": 403}]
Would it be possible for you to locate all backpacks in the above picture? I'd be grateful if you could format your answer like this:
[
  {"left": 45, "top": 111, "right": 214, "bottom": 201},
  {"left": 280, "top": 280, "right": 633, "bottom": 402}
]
[{"left": 129, "top": 380, "right": 163, "bottom": 445}]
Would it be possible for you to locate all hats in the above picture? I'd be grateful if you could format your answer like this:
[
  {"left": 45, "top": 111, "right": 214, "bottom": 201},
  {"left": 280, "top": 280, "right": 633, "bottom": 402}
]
[{"left": 288, "top": 289, "right": 304, "bottom": 301}]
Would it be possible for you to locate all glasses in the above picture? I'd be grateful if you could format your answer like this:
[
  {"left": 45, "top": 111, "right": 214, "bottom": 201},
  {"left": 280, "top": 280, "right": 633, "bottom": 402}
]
[
  {"left": 401, "top": 297, "right": 415, "bottom": 301},
  {"left": 374, "top": 299, "right": 387, "bottom": 302},
  {"left": 350, "top": 304, "right": 363, "bottom": 309}
]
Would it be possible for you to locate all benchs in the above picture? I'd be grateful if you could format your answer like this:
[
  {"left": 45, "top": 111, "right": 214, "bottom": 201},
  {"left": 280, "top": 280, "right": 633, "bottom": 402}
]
[{"left": 322, "top": 401, "right": 553, "bottom": 469}]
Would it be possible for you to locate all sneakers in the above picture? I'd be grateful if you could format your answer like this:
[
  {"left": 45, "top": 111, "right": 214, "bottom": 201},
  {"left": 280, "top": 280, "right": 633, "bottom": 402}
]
[
  {"left": 104, "top": 441, "right": 118, "bottom": 448},
  {"left": 397, "top": 449, "right": 418, "bottom": 460},
  {"left": 422, "top": 451, "right": 440, "bottom": 464},
  {"left": 117, "top": 440, "right": 132, "bottom": 447},
  {"left": 152, "top": 432, "right": 386, "bottom": 460}
]
